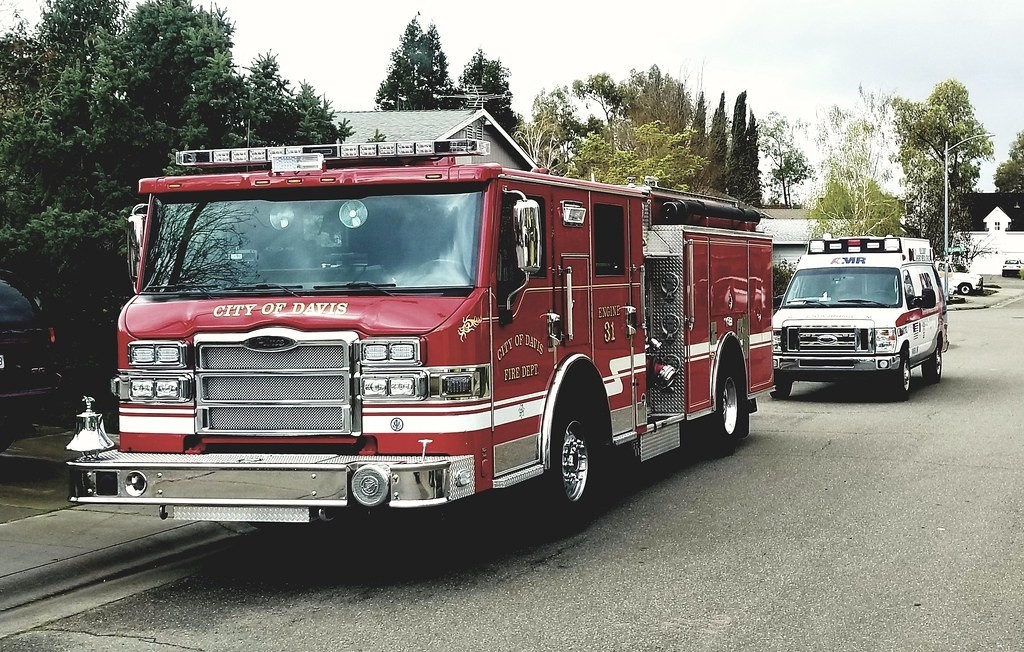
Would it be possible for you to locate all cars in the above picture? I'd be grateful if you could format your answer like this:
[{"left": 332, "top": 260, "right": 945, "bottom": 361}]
[
  {"left": 1002, "top": 259, "right": 1024, "bottom": 278},
  {"left": 0, "top": 259, "right": 76, "bottom": 452}
]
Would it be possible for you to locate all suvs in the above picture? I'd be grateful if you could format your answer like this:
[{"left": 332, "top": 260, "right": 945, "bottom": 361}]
[
  {"left": 770, "top": 233, "right": 949, "bottom": 401},
  {"left": 934, "top": 260, "right": 983, "bottom": 295}
]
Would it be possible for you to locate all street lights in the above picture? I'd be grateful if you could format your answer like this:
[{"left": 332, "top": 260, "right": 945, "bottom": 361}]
[{"left": 944, "top": 133, "right": 995, "bottom": 303}]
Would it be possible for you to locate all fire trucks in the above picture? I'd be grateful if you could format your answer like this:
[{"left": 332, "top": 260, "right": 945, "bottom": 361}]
[{"left": 65, "top": 137, "right": 777, "bottom": 553}]
